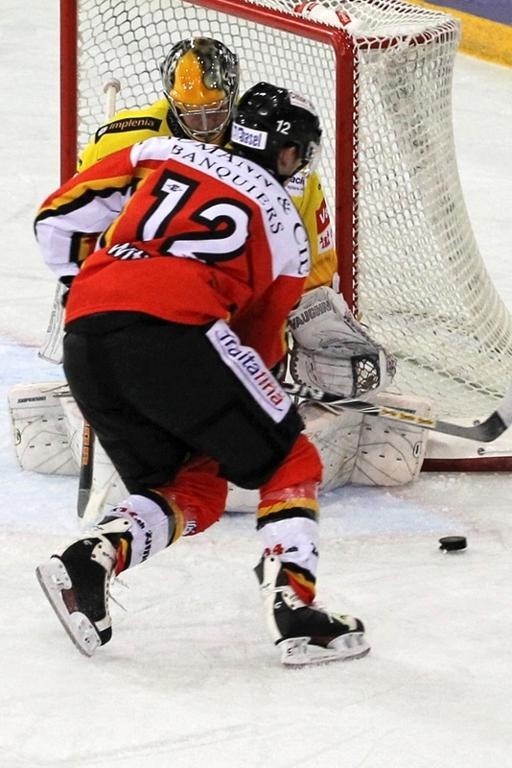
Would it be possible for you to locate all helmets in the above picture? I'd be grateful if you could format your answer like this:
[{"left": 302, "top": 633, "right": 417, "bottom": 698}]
[
  {"left": 229, "top": 81, "right": 322, "bottom": 171},
  {"left": 160, "top": 35, "right": 241, "bottom": 108}
]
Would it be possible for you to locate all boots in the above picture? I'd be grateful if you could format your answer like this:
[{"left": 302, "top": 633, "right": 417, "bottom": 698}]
[
  {"left": 59, "top": 515, "right": 134, "bottom": 648},
  {"left": 253, "top": 555, "right": 366, "bottom": 646}
]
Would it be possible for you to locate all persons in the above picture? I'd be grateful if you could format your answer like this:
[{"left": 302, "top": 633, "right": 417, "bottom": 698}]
[
  {"left": 6, "top": 36, "right": 434, "bottom": 515},
  {"left": 32, "top": 81, "right": 365, "bottom": 649}
]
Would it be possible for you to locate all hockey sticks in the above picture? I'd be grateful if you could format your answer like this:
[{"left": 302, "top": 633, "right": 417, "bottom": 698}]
[{"left": 292, "top": 387, "right": 511, "bottom": 443}]
